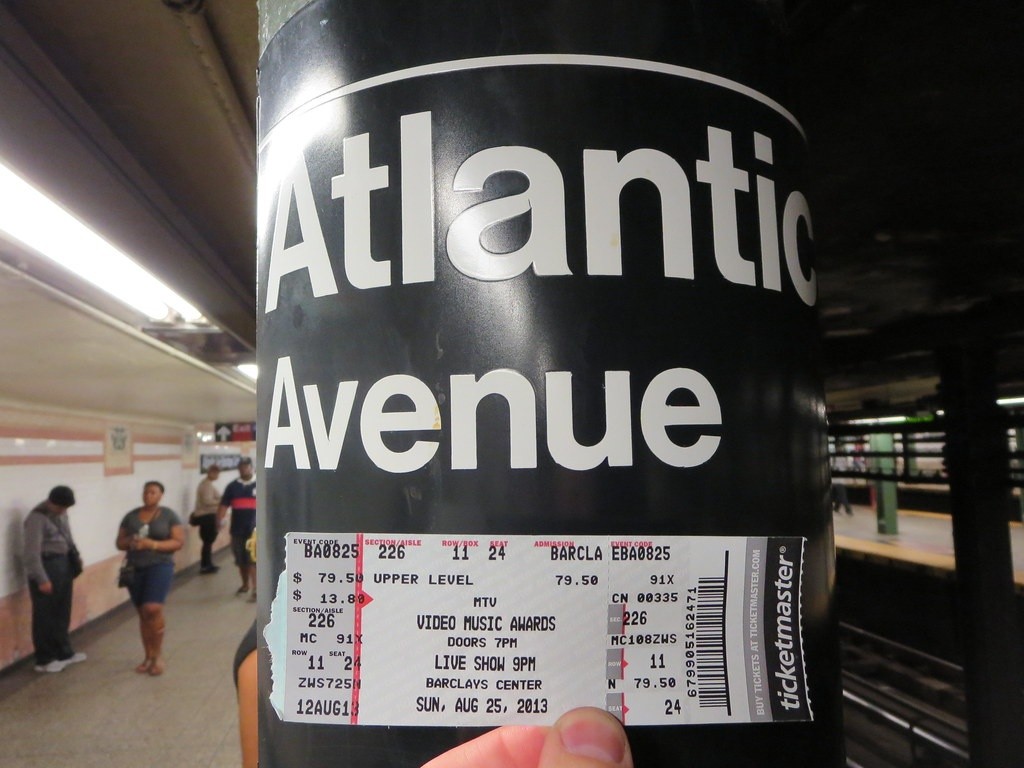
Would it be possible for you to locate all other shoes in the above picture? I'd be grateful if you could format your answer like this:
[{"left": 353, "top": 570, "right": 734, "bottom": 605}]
[
  {"left": 237, "top": 583, "right": 248, "bottom": 595},
  {"left": 247, "top": 593, "right": 256, "bottom": 603},
  {"left": 199, "top": 565, "right": 219, "bottom": 576}
]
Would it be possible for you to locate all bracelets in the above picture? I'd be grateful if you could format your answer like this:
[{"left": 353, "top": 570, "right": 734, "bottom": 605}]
[{"left": 152, "top": 541, "right": 157, "bottom": 550}]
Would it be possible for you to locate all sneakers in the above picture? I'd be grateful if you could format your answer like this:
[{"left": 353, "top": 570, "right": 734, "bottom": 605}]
[
  {"left": 58, "top": 653, "right": 86, "bottom": 664},
  {"left": 34, "top": 659, "right": 63, "bottom": 672}
]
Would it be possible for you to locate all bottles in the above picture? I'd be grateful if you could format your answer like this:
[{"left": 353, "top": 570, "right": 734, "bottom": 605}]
[{"left": 139, "top": 525, "right": 149, "bottom": 538}]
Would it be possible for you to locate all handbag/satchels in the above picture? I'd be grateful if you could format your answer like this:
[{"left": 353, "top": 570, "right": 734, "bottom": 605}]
[
  {"left": 118, "top": 566, "right": 139, "bottom": 588},
  {"left": 68, "top": 549, "right": 82, "bottom": 579},
  {"left": 190, "top": 512, "right": 201, "bottom": 526}
]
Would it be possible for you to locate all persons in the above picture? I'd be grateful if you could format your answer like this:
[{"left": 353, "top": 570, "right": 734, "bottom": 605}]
[
  {"left": 831, "top": 442, "right": 852, "bottom": 513},
  {"left": 22, "top": 486, "right": 86, "bottom": 671},
  {"left": 193, "top": 466, "right": 222, "bottom": 573},
  {"left": 215, "top": 456, "right": 257, "bottom": 603},
  {"left": 420, "top": 707, "right": 632, "bottom": 768},
  {"left": 114, "top": 480, "right": 183, "bottom": 676}
]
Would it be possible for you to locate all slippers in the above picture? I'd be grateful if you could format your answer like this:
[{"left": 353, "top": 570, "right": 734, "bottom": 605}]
[
  {"left": 137, "top": 662, "right": 149, "bottom": 671},
  {"left": 150, "top": 663, "right": 162, "bottom": 675}
]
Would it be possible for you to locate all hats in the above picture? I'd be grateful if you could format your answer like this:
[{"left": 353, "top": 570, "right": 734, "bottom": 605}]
[
  {"left": 236, "top": 456, "right": 252, "bottom": 468},
  {"left": 50, "top": 485, "right": 74, "bottom": 506}
]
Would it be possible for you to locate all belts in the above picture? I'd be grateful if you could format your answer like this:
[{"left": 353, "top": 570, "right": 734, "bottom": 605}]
[{"left": 41, "top": 554, "right": 69, "bottom": 561}]
